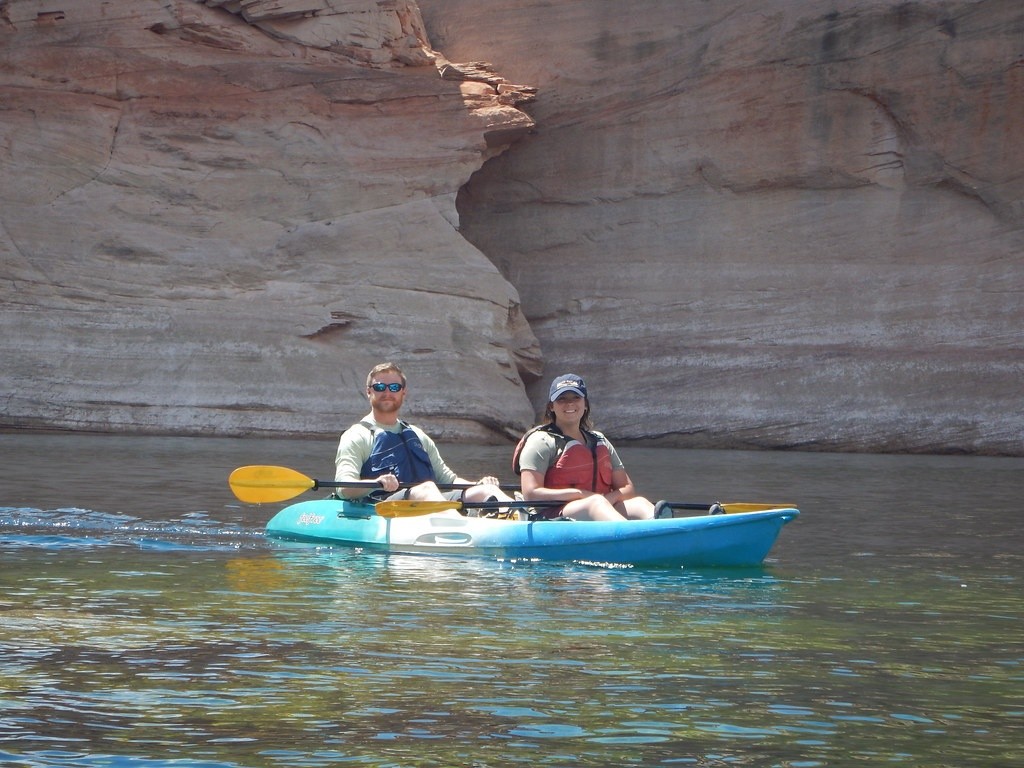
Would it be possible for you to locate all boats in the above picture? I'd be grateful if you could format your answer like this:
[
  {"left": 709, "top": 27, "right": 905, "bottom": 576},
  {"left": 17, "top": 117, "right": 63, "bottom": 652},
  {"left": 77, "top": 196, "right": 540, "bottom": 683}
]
[{"left": 264, "top": 483, "right": 801, "bottom": 574}]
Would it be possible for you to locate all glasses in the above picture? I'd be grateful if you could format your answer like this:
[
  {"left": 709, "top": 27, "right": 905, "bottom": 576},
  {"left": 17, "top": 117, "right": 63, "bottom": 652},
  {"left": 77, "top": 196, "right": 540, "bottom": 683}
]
[{"left": 370, "top": 382, "right": 404, "bottom": 392}]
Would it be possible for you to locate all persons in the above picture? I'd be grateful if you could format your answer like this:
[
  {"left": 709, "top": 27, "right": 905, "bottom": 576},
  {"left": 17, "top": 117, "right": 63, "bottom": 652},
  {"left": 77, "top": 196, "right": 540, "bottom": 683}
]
[
  {"left": 335, "top": 361, "right": 520, "bottom": 517},
  {"left": 519, "top": 373, "right": 675, "bottom": 521}
]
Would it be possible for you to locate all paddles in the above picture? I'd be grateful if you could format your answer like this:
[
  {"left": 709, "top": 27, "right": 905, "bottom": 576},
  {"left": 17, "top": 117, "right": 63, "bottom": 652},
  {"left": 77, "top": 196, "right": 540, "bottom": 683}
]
[
  {"left": 227, "top": 464, "right": 521, "bottom": 504},
  {"left": 374, "top": 500, "right": 799, "bottom": 520}
]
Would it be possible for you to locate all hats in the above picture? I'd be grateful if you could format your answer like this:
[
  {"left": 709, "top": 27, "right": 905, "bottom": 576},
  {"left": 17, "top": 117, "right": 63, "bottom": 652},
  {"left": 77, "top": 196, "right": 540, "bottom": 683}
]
[{"left": 550, "top": 373, "right": 586, "bottom": 402}]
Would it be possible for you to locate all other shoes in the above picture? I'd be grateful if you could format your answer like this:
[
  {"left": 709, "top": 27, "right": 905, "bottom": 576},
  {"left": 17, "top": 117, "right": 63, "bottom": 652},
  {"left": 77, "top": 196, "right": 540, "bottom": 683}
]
[
  {"left": 479, "top": 495, "right": 499, "bottom": 519},
  {"left": 709, "top": 504, "right": 725, "bottom": 515},
  {"left": 654, "top": 500, "right": 674, "bottom": 519}
]
